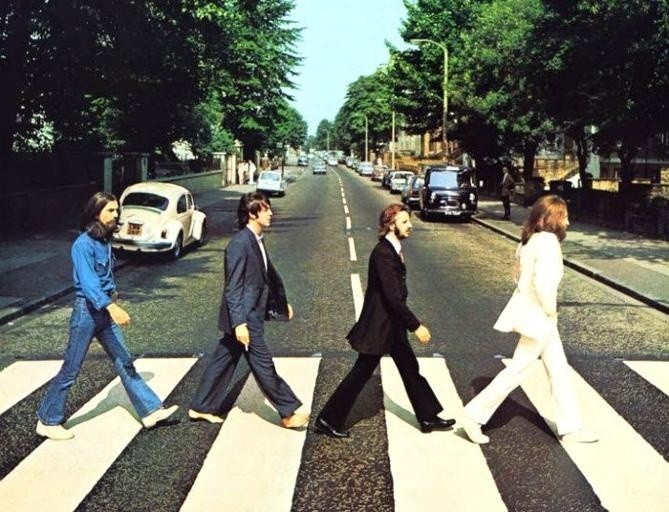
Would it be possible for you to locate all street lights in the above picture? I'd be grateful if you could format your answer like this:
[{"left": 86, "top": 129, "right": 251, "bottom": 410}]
[
  {"left": 322, "top": 126, "right": 331, "bottom": 154},
  {"left": 353, "top": 111, "right": 371, "bottom": 163},
  {"left": 408, "top": 36, "right": 450, "bottom": 144}
]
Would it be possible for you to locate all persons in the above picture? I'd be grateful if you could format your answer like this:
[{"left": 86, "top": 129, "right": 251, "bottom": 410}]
[
  {"left": 36, "top": 191, "right": 180, "bottom": 441},
  {"left": 187, "top": 189, "right": 311, "bottom": 430},
  {"left": 456, "top": 193, "right": 598, "bottom": 444},
  {"left": 499, "top": 166, "right": 515, "bottom": 220},
  {"left": 315, "top": 203, "right": 457, "bottom": 437}
]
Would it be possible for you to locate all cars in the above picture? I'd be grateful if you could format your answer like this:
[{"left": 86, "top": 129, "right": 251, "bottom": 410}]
[
  {"left": 254, "top": 145, "right": 486, "bottom": 222},
  {"left": 112, "top": 180, "right": 209, "bottom": 264}
]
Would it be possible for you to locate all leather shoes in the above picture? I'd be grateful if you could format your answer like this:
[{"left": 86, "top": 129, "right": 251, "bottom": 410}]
[
  {"left": 142, "top": 405, "right": 179, "bottom": 430},
  {"left": 315, "top": 417, "right": 348, "bottom": 438},
  {"left": 282, "top": 414, "right": 308, "bottom": 428},
  {"left": 562, "top": 431, "right": 598, "bottom": 443},
  {"left": 453, "top": 411, "right": 490, "bottom": 445},
  {"left": 188, "top": 409, "right": 223, "bottom": 423},
  {"left": 35, "top": 421, "right": 74, "bottom": 441},
  {"left": 420, "top": 418, "right": 456, "bottom": 433}
]
